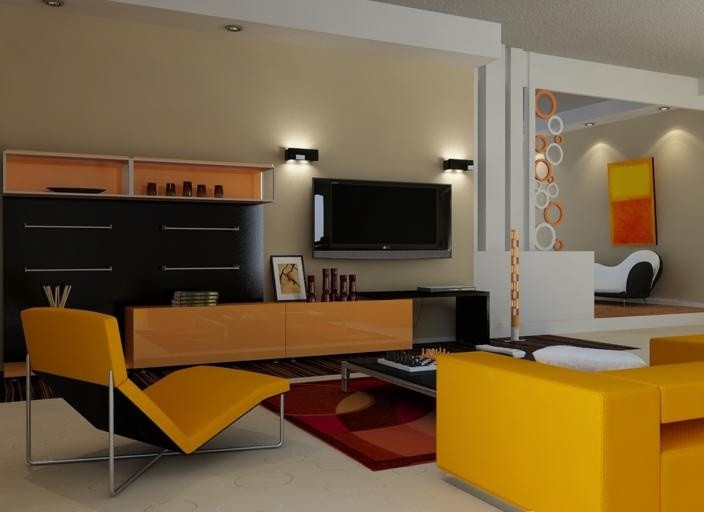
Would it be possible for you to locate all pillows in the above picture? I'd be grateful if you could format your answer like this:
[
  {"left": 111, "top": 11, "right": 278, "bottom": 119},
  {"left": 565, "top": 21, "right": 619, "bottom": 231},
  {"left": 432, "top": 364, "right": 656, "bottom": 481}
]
[{"left": 531, "top": 344, "right": 648, "bottom": 371}]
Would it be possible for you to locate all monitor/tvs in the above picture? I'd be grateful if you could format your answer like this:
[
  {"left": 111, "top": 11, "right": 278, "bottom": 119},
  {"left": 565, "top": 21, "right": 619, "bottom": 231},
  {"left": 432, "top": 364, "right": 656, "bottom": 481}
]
[{"left": 313, "top": 176, "right": 451, "bottom": 260}]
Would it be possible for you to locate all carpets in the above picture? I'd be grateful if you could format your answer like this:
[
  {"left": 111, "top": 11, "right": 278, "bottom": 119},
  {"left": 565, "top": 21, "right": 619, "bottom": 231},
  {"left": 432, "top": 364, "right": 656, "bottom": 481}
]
[{"left": 261, "top": 378, "right": 436, "bottom": 471}]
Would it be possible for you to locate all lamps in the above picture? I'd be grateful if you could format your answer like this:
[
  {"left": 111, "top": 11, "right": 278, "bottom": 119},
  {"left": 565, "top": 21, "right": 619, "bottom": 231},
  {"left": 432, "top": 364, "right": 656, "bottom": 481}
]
[
  {"left": 286, "top": 148, "right": 318, "bottom": 162},
  {"left": 444, "top": 159, "right": 474, "bottom": 172}
]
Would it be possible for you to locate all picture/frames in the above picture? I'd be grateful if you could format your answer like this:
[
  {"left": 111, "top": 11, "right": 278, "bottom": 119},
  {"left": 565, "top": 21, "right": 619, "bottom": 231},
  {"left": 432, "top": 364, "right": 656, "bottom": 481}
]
[{"left": 271, "top": 255, "right": 308, "bottom": 302}]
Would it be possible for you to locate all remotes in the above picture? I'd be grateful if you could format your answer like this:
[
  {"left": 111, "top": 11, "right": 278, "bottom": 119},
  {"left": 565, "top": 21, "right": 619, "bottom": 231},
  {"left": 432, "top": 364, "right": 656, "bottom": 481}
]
[{"left": 475, "top": 344, "right": 526, "bottom": 358}]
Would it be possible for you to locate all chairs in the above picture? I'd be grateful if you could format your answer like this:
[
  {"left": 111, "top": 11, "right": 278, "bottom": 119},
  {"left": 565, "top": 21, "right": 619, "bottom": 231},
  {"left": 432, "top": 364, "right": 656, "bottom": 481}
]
[
  {"left": 20, "top": 307, "right": 290, "bottom": 496},
  {"left": 595, "top": 249, "right": 663, "bottom": 308}
]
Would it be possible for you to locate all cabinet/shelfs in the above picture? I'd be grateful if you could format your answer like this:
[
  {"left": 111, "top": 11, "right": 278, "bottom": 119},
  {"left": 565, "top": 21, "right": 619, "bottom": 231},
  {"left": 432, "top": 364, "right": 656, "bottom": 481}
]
[
  {"left": 123, "top": 289, "right": 490, "bottom": 370},
  {"left": 4, "top": 150, "right": 275, "bottom": 378}
]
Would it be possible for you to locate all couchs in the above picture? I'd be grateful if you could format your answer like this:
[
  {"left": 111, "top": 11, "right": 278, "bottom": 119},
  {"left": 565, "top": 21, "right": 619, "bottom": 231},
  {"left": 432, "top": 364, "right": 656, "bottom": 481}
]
[{"left": 436, "top": 335, "right": 704, "bottom": 511}]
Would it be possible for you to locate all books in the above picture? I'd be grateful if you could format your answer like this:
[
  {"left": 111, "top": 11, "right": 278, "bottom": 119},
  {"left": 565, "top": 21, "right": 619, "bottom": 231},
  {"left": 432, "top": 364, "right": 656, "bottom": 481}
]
[{"left": 171, "top": 291, "right": 219, "bottom": 307}]
